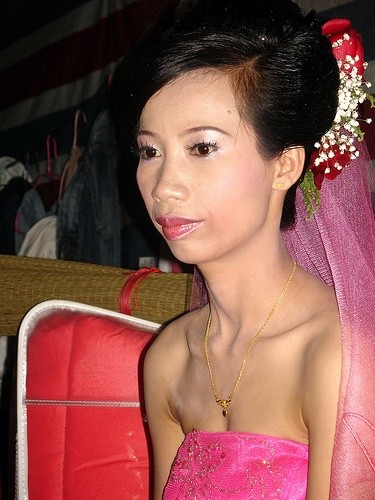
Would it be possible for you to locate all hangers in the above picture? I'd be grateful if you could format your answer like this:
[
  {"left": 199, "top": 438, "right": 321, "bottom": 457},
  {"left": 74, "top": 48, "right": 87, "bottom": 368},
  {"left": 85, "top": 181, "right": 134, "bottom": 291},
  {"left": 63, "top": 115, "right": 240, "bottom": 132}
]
[{"left": 22, "top": 108, "right": 88, "bottom": 202}]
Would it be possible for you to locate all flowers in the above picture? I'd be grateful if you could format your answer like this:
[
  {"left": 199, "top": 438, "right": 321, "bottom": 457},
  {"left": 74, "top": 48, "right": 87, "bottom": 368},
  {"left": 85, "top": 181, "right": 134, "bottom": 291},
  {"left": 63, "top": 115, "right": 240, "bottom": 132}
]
[{"left": 299, "top": 19, "right": 375, "bottom": 219}]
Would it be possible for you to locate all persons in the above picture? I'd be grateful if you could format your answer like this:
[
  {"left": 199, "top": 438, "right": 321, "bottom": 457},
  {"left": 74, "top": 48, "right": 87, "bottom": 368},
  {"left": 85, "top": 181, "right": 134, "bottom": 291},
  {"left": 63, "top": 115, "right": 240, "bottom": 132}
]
[{"left": 131, "top": 0, "right": 375, "bottom": 500}]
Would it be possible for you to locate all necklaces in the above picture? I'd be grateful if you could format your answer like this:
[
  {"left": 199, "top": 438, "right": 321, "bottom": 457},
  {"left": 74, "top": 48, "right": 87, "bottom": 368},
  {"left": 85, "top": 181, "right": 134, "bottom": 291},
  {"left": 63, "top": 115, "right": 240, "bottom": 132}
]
[{"left": 203, "top": 262, "right": 297, "bottom": 417}]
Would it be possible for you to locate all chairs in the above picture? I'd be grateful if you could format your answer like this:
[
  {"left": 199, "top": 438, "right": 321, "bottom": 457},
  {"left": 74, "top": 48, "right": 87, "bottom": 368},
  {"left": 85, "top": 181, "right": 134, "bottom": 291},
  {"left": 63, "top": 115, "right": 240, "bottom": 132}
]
[{"left": 17, "top": 297, "right": 172, "bottom": 500}]
